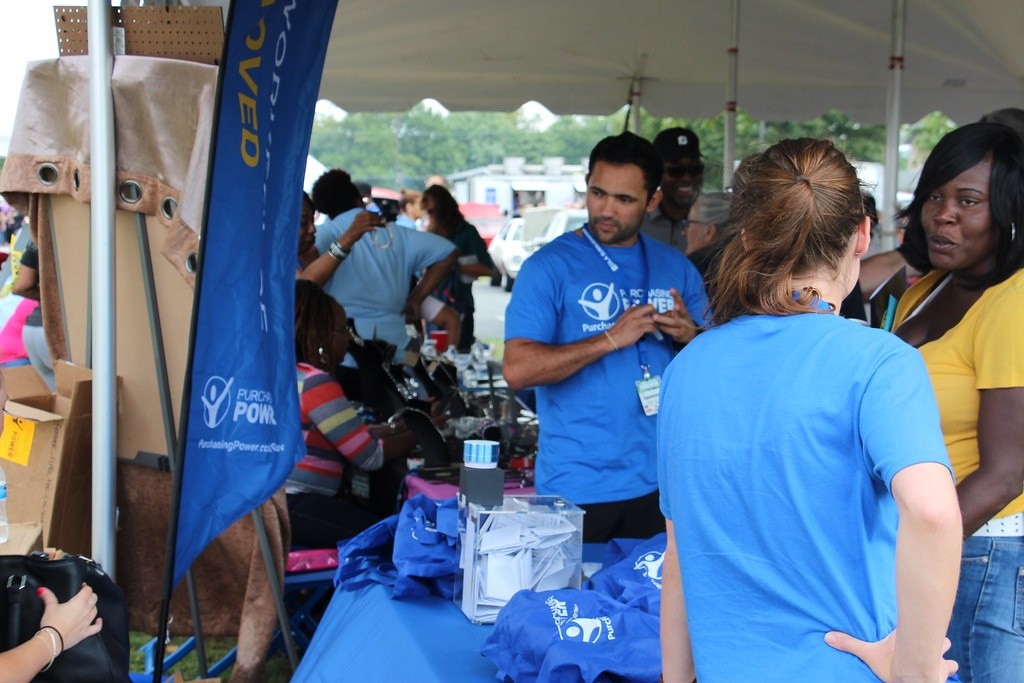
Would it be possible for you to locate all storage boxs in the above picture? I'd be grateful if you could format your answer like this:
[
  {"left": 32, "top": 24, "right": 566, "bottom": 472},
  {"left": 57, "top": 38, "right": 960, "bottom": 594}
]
[
  {"left": 0, "top": 359, "right": 122, "bottom": 558},
  {"left": 453, "top": 494, "right": 586, "bottom": 624}
]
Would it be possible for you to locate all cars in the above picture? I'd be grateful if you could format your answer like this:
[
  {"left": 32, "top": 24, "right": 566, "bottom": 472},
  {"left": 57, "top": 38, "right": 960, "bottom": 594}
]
[
  {"left": 849, "top": 160, "right": 900, "bottom": 260},
  {"left": 486, "top": 204, "right": 562, "bottom": 293},
  {"left": 895, "top": 170, "right": 922, "bottom": 245},
  {"left": 521, "top": 206, "right": 589, "bottom": 258}
]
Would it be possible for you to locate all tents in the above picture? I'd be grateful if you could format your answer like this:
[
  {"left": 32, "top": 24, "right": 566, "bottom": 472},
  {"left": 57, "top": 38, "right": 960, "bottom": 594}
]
[{"left": 85, "top": 0, "right": 1024, "bottom": 580}]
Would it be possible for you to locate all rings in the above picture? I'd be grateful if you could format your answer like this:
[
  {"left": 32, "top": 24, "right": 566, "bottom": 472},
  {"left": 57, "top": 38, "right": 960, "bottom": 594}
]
[{"left": 88, "top": 601, "right": 95, "bottom": 607}]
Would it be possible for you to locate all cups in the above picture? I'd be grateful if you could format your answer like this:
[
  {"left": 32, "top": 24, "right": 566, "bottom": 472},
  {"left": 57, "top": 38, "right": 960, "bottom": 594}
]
[{"left": 430, "top": 330, "right": 448, "bottom": 354}]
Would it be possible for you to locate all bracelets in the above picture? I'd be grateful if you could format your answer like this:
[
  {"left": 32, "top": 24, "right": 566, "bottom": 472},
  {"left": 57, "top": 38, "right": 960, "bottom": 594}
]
[
  {"left": 604, "top": 330, "right": 618, "bottom": 351},
  {"left": 327, "top": 239, "right": 350, "bottom": 261},
  {"left": 389, "top": 422, "right": 396, "bottom": 434},
  {"left": 31, "top": 626, "right": 64, "bottom": 672}
]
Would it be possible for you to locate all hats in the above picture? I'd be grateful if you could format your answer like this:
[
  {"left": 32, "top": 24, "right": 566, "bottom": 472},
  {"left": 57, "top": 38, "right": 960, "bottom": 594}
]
[
  {"left": 480, "top": 532, "right": 667, "bottom": 683},
  {"left": 653, "top": 127, "right": 700, "bottom": 157}
]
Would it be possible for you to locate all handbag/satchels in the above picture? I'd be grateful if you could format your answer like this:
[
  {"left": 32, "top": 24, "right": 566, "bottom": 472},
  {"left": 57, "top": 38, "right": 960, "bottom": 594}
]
[
  {"left": 0, "top": 555, "right": 133, "bottom": 683},
  {"left": 333, "top": 493, "right": 459, "bottom": 601}
]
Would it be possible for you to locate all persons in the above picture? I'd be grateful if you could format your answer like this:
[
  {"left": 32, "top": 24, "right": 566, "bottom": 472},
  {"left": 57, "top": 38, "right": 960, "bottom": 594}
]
[
  {"left": 0, "top": 548, "right": 103, "bottom": 683},
  {"left": 499, "top": 130, "right": 717, "bottom": 543},
  {"left": 286, "top": 165, "right": 500, "bottom": 555},
  {"left": 13, "top": 235, "right": 54, "bottom": 395},
  {"left": 643, "top": 107, "right": 1024, "bottom": 682}
]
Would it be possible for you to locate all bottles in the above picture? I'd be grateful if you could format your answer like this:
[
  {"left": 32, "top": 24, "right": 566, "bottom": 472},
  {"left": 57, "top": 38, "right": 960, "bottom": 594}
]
[{"left": 0, "top": 466, "right": 8, "bottom": 544}]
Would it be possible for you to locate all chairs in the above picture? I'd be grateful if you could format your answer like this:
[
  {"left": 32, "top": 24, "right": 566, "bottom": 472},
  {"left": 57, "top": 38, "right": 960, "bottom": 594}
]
[{"left": 250, "top": 504, "right": 338, "bottom": 683}]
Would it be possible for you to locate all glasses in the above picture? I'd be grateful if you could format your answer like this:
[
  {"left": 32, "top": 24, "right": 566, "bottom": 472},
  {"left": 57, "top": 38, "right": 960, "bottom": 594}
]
[
  {"left": 333, "top": 326, "right": 350, "bottom": 335},
  {"left": 662, "top": 161, "right": 705, "bottom": 178},
  {"left": 682, "top": 219, "right": 710, "bottom": 231}
]
